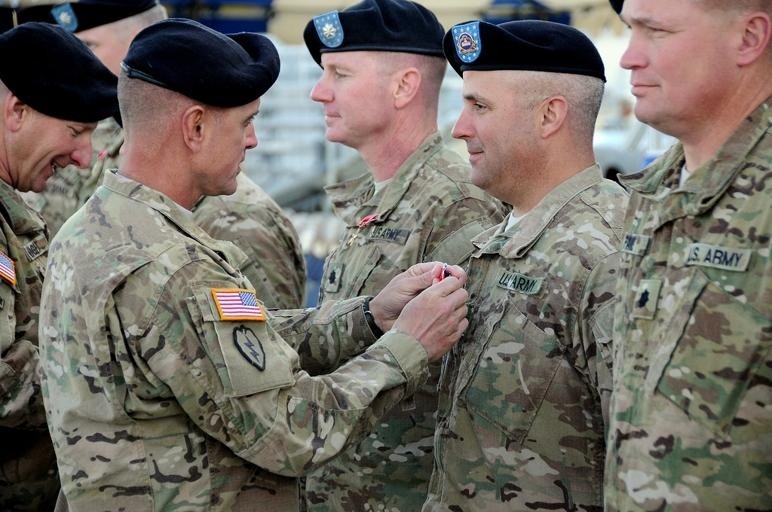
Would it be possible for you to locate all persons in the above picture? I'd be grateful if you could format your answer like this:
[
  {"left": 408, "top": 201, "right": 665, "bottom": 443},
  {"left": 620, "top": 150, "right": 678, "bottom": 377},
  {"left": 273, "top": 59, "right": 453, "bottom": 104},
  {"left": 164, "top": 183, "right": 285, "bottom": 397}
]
[
  {"left": 412, "top": 19, "right": 635, "bottom": 511},
  {"left": 605, "top": 1, "right": 772, "bottom": 512},
  {"left": 301, "top": 0, "right": 518, "bottom": 512},
  {"left": 36, "top": 2, "right": 308, "bottom": 321},
  {"left": 2, "top": 22, "right": 117, "bottom": 512},
  {"left": 38, "top": 12, "right": 472, "bottom": 512}
]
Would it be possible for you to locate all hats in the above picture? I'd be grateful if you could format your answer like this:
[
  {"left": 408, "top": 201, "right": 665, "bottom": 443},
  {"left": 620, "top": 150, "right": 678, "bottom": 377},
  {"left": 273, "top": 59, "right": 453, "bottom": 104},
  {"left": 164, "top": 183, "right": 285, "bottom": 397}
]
[
  {"left": 444, "top": 20, "right": 606, "bottom": 82},
  {"left": 121, "top": 17, "right": 280, "bottom": 107},
  {"left": 304, "top": 1, "right": 444, "bottom": 70},
  {"left": 1, "top": 22, "right": 117, "bottom": 122}
]
